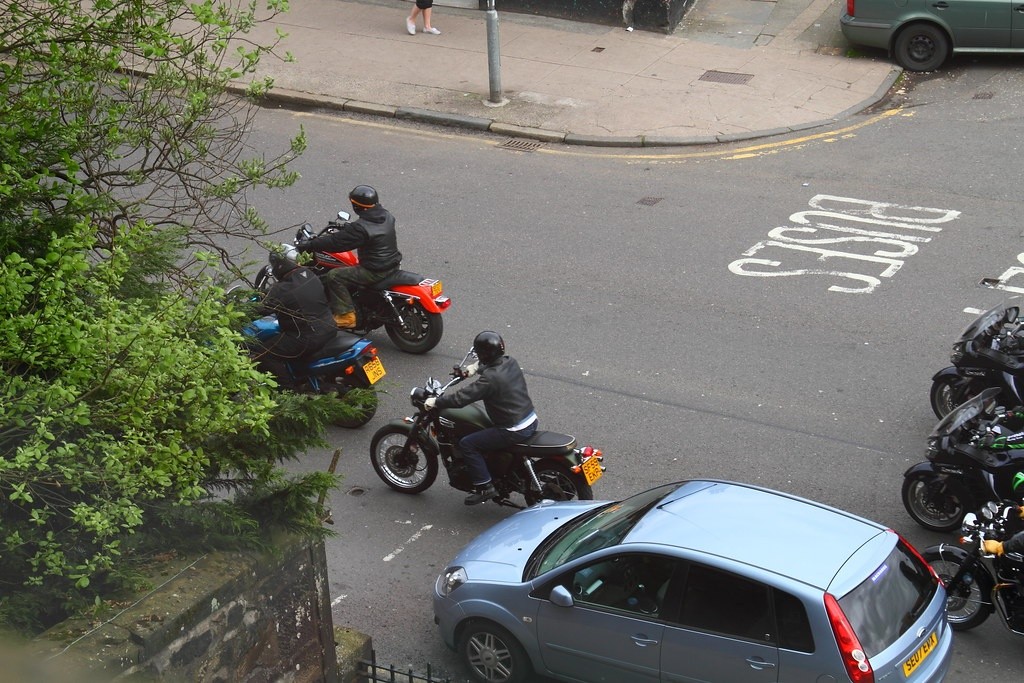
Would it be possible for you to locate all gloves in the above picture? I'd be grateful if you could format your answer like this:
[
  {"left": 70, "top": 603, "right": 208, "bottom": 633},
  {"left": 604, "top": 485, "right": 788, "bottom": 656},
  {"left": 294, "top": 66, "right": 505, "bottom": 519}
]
[
  {"left": 344, "top": 222, "right": 351, "bottom": 229},
  {"left": 297, "top": 242, "right": 313, "bottom": 256},
  {"left": 983, "top": 540, "right": 1004, "bottom": 557},
  {"left": 1012, "top": 406, "right": 1024, "bottom": 416},
  {"left": 466, "top": 361, "right": 479, "bottom": 378},
  {"left": 979, "top": 434, "right": 1007, "bottom": 449},
  {"left": 1020, "top": 506, "right": 1024, "bottom": 517},
  {"left": 424, "top": 398, "right": 436, "bottom": 411}
]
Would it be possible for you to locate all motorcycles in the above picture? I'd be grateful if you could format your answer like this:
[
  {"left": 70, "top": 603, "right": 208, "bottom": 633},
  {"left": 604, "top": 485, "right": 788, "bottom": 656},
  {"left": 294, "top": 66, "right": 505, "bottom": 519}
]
[
  {"left": 370, "top": 347, "right": 607, "bottom": 511},
  {"left": 186, "top": 265, "right": 386, "bottom": 429},
  {"left": 902, "top": 291, "right": 1024, "bottom": 634},
  {"left": 255, "top": 211, "right": 450, "bottom": 355}
]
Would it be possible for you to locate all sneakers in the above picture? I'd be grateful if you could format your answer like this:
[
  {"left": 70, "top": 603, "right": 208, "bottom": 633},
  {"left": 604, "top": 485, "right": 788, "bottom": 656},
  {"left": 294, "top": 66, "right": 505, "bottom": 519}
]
[
  {"left": 406, "top": 17, "right": 416, "bottom": 34},
  {"left": 423, "top": 27, "right": 441, "bottom": 35}
]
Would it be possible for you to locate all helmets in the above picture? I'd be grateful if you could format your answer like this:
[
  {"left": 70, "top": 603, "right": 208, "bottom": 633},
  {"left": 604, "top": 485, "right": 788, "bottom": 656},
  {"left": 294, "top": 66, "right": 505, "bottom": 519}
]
[
  {"left": 473, "top": 331, "right": 505, "bottom": 365},
  {"left": 349, "top": 185, "right": 379, "bottom": 208},
  {"left": 268, "top": 244, "right": 301, "bottom": 281}
]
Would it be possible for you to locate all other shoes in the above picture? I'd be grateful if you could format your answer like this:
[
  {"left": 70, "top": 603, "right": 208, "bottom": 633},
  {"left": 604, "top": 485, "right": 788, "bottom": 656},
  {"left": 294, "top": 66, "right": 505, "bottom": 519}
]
[{"left": 333, "top": 313, "right": 356, "bottom": 327}]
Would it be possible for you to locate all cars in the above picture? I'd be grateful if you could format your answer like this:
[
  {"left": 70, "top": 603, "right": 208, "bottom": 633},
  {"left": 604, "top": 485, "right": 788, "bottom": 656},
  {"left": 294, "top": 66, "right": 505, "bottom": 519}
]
[
  {"left": 433, "top": 478, "right": 953, "bottom": 683},
  {"left": 839, "top": 0, "right": 1024, "bottom": 72}
]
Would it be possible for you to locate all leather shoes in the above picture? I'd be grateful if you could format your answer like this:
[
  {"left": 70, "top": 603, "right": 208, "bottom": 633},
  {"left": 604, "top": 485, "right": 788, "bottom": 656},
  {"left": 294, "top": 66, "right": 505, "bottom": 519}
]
[{"left": 464, "top": 487, "right": 499, "bottom": 505}]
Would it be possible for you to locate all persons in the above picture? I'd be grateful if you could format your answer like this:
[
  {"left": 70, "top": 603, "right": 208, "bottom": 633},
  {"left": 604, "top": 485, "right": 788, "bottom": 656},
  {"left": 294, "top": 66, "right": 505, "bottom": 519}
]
[
  {"left": 424, "top": 331, "right": 538, "bottom": 506},
  {"left": 968, "top": 319, "right": 1024, "bottom": 636},
  {"left": 237, "top": 242, "right": 337, "bottom": 393},
  {"left": 294, "top": 185, "right": 403, "bottom": 328},
  {"left": 405, "top": 0, "right": 442, "bottom": 35}
]
